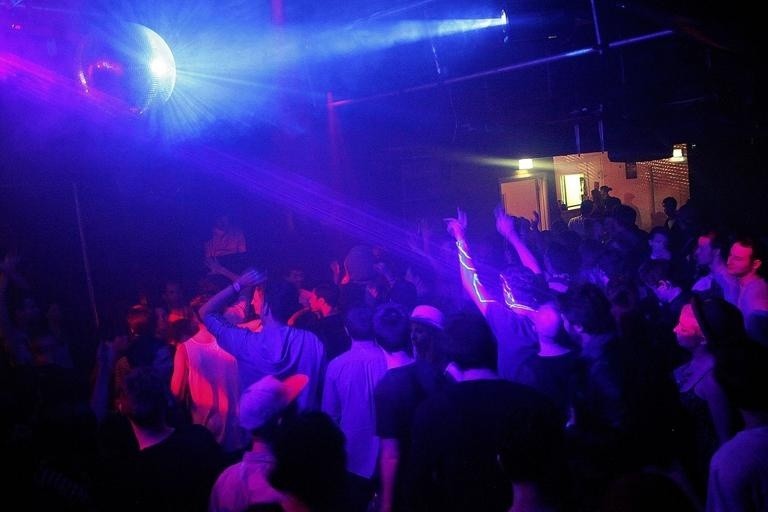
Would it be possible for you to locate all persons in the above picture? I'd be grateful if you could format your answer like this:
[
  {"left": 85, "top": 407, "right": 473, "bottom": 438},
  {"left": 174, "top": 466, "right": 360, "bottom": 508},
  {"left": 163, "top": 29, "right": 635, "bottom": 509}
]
[{"left": 2, "top": 185, "right": 768, "bottom": 512}]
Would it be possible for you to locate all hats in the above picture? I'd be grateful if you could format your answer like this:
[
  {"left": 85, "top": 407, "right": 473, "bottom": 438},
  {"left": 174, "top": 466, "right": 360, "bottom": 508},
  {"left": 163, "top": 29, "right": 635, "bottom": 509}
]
[
  {"left": 237, "top": 372, "right": 311, "bottom": 430},
  {"left": 406, "top": 305, "right": 447, "bottom": 335}
]
